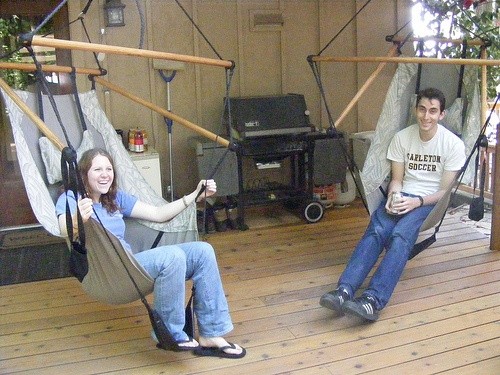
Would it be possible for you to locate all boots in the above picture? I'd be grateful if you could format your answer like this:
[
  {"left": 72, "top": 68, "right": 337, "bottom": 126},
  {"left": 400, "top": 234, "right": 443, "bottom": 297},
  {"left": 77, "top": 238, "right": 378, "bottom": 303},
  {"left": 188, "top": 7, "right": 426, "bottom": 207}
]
[
  {"left": 227, "top": 200, "right": 239, "bottom": 229},
  {"left": 196, "top": 209, "right": 207, "bottom": 235},
  {"left": 212, "top": 202, "right": 228, "bottom": 232},
  {"left": 206, "top": 209, "right": 216, "bottom": 235}
]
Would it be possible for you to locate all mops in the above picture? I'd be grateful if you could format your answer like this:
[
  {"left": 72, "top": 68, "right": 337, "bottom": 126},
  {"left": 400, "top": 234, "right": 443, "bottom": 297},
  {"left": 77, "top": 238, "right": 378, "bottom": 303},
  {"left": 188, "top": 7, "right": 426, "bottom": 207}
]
[{"left": 152, "top": 57, "right": 186, "bottom": 202}]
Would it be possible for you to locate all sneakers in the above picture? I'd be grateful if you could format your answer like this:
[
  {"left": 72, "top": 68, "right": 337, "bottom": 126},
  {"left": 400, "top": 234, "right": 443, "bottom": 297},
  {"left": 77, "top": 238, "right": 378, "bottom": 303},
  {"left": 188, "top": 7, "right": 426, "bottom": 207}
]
[
  {"left": 342, "top": 296, "right": 379, "bottom": 321},
  {"left": 319, "top": 288, "right": 350, "bottom": 311}
]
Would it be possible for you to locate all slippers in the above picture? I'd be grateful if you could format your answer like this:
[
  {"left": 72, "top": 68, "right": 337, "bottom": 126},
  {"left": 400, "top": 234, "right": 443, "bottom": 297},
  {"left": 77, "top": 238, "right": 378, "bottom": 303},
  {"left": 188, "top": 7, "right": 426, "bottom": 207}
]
[
  {"left": 194, "top": 342, "right": 246, "bottom": 359},
  {"left": 156, "top": 341, "right": 200, "bottom": 350}
]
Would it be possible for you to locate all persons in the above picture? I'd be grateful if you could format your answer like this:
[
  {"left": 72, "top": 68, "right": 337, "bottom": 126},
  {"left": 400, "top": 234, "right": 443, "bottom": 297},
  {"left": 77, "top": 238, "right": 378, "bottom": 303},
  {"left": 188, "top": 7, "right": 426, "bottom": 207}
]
[
  {"left": 320, "top": 88, "right": 467, "bottom": 323},
  {"left": 54, "top": 149, "right": 247, "bottom": 359}
]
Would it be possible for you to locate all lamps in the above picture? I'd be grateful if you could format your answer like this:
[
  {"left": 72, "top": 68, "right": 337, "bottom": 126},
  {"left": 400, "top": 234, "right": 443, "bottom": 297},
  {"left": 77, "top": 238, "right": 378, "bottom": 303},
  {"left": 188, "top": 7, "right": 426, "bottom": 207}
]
[{"left": 104, "top": 0, "right": 126, "bottom": 27}]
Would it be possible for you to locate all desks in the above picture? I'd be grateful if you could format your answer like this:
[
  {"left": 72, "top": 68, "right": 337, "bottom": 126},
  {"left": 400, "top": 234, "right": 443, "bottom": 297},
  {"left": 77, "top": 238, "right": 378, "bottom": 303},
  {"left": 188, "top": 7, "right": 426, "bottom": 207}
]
[{"left": 128, "top": 145, "right": 162, "bottom": 198}]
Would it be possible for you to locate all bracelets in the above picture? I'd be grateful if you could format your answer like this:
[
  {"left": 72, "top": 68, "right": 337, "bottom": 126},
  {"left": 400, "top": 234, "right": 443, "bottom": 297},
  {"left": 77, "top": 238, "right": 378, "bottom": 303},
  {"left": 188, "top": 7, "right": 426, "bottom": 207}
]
[
  {"left": 183, "top": 196, "right": 188, "bottom": 207},
  {"left": 418, "top": 196, "right": 424, "bottom": 207}
]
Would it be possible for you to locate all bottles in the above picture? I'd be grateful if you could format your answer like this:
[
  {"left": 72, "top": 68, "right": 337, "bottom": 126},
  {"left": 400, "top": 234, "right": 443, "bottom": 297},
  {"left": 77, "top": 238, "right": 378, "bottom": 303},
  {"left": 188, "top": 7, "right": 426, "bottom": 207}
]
[{"left": 127, "top": 128, "right": 149, "bottom": 153}]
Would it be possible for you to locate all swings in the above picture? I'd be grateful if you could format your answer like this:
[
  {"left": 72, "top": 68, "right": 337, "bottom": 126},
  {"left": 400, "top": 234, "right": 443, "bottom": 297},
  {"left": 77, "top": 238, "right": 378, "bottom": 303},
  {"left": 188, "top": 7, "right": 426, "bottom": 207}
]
[
  {"left": 0, "top": 0, "right": 239, "bottom": 304},
  {"left": 307, "top": 0, "right": 500, "bottom": 260}
]
